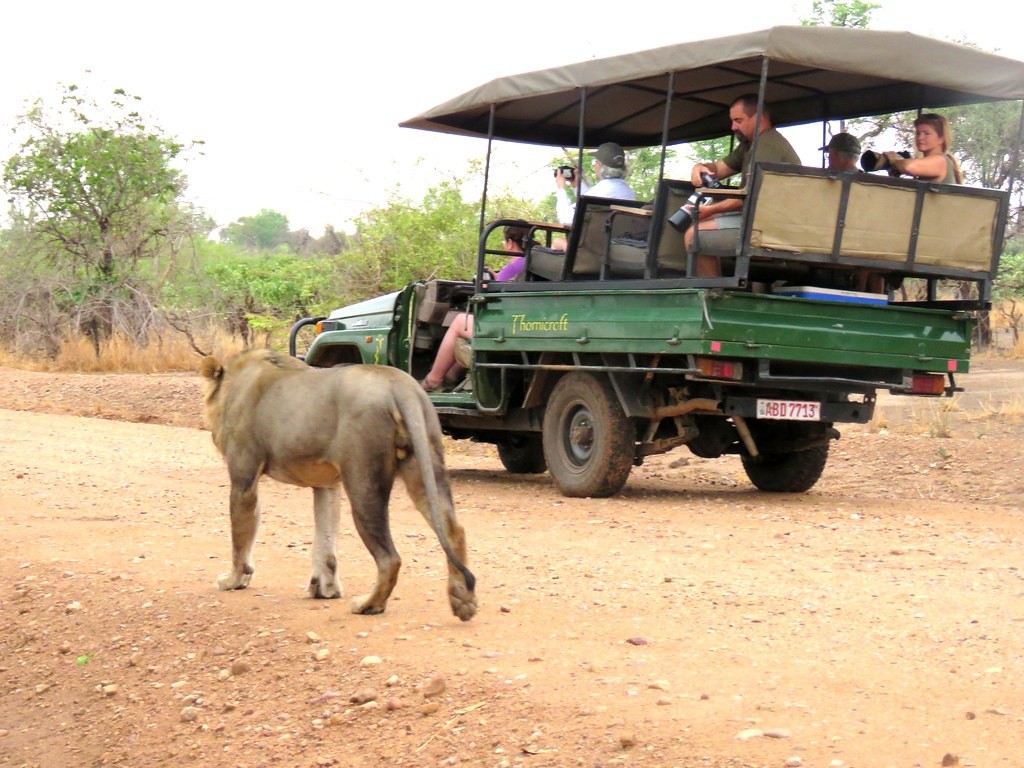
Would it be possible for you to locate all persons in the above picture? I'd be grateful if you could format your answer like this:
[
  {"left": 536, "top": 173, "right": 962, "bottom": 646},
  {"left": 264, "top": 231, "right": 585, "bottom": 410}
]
[
  {"left": 846, "top": 113, "right": 964, "bottom": 295},
  {"left": 416, "top": 218, "right": 540, "bottom": 394},
  {"left": 550, "top": 142, "right": 638, "bottom": 256},
  {"left": 683, "top": 93, "right": 803, "bottom": 295},
  {"left": 817, "top": 133, "right": 863, "bottom": 174}
]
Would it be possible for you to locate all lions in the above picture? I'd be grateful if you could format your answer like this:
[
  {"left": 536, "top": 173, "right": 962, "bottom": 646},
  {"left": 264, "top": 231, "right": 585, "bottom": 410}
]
[{"left": 197, "top": 348, "right": 480, "bottom": 624}]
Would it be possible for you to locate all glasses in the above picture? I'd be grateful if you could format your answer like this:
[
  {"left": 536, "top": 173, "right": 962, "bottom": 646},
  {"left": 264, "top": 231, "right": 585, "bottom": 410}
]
[{"left": 825, "top": 151, "right": 830, "bottom": 159}]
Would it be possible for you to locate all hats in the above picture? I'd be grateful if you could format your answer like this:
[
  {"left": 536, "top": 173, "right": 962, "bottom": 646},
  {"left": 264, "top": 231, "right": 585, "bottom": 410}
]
[
  {"left": 588, "top": 142, "right": 624, "bottom": 168},
  {"left": 818, "top": 133, "right": 861, "bottom": 154}
]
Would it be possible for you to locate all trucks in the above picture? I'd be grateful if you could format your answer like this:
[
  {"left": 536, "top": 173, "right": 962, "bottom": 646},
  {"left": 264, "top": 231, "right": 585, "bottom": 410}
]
[{"left": 282, "top": 24, "right": 1024, "bottom": 501}]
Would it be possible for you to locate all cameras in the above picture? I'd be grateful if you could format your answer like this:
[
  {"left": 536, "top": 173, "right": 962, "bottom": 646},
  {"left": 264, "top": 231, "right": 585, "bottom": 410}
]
[
  {"left": 861, "top": 151, "right": 911, "bottom": 177},
  {"left": 668, "top": 172, "right": 725, "bottom": 233},
  {"left": 473, "top": 269, "right": 494, "bottom": 284},
  {"left": 554, "top": 166, "right": 575, "bottom": 181}
]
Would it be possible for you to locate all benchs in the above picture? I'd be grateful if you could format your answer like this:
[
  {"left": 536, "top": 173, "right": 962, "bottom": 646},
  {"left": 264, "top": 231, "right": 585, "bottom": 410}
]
[
  {"left": 599, "top": 178, "right": 740, "bottom": 280},
  {"left": 685, "top": 160, "right": 1011, "bottom": 313},
  {"left": 524, "top": 195, "right": 655, "bottom": 282}
]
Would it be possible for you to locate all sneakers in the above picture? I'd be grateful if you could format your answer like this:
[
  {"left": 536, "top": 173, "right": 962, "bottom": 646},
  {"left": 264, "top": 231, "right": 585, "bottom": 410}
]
[
  {"left": 438, "top": 378, "right": 461, "bottom": 393},
  {"left": 420, "top": 376, "right": 445, "bottom": 393}
]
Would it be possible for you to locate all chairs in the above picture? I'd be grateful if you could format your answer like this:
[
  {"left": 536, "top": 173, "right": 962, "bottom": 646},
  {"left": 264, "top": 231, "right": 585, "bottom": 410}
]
[{"left": 451, "top": 271, "right": 527, "bottom": 392}]
